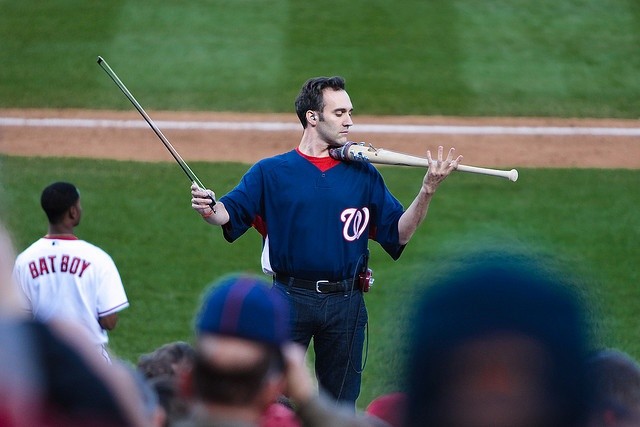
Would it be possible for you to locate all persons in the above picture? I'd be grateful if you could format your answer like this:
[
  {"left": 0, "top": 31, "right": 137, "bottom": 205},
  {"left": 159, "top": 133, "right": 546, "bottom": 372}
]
[
  {"left": 367, "top": 250, "right": 639, "bottom": 427},
  {"left": 165, "top": 272, "right": 386, "bottom": 427},
  {"left": 135, "top": 340, "right": 199, "bottom": 427},
  {"left": 191, "top": 76, "right": 464, "bottom": 415},
  {"left": 13, "top": 183, "right": 129, "bottom": 365},
  {"left": 583, "top": 345, "right": 639, "bottom": 427},
  {"left": 1, "top": 222, "right": 163, "bottom": 427}
]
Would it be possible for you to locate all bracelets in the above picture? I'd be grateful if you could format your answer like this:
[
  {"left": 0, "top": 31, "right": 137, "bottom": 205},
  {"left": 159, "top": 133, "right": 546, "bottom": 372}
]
[{"left": 200, "top": 210, "right": 215, "bottom": 219}]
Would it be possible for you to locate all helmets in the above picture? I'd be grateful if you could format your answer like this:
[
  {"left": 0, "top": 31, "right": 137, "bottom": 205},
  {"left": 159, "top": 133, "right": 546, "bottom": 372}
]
[{"left": 198, "top": 276, "right": 291, "bottom": 345}]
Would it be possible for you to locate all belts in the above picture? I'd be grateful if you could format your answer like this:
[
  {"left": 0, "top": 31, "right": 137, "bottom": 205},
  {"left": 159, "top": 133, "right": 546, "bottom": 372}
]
[{"left": 276, "top": 276, "right": 360, "bottom": 295}]
